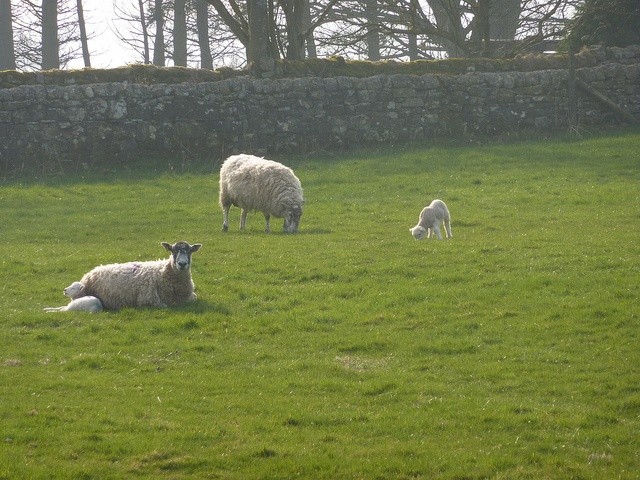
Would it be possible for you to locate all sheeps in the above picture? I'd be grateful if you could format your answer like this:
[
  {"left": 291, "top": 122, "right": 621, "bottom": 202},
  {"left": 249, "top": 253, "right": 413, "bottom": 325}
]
[
  {"left": 80, "top": 241, "right": 202, "bottom": 312},
  {"left": 409, "top": 199, "right": 453, "bottom": 241},
  {"left": 44, "top": 282, "right": 103, "bottom": 313},
  {"left": 219, "top": 153, "right": 305, "bottom": 234}
]
